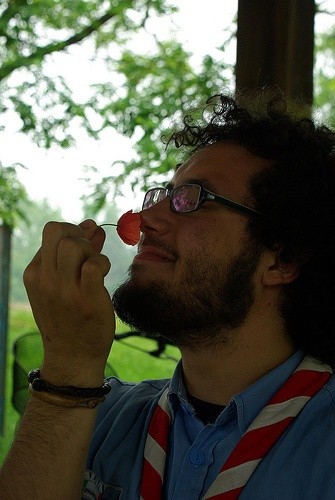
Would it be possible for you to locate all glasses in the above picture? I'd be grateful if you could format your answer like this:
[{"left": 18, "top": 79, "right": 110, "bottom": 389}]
[{"left": 142, "top": 182, "right": 271, "bottom": 226}]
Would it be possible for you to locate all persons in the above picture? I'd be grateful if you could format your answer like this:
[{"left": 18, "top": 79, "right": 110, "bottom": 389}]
[{"left": 0, "top": 90, "right": 334, "bottom": 500}]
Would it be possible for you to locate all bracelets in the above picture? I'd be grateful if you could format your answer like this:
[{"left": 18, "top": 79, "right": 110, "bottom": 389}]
[{"left": 27, "top": 368, "right": 110, "bottom": 411}]
